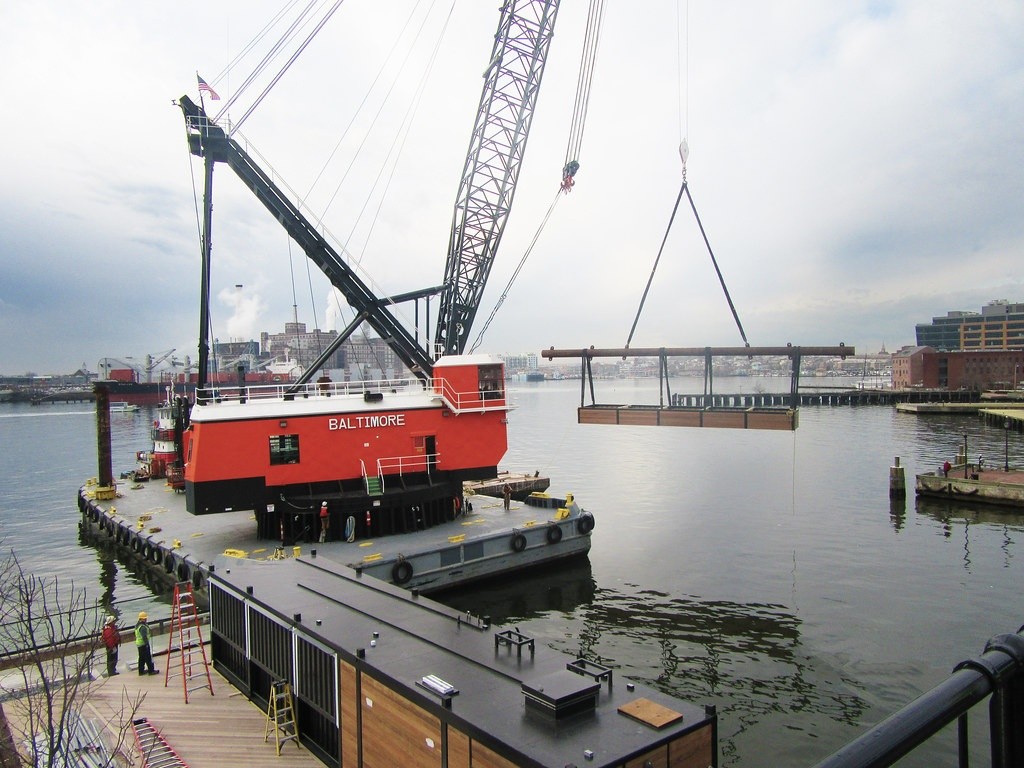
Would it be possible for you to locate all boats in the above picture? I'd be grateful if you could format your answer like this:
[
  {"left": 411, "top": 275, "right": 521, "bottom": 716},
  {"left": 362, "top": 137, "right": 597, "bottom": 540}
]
[{"left": 110, "top": 401, "right": 141, "bottom": 412}]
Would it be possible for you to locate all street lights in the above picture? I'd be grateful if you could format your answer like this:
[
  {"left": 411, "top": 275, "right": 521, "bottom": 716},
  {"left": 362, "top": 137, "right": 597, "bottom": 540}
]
[
  {"left": 961, "top": 426, "right": 968, "bottom": 479},
  {"left": 1003, "top": 418, "right": 1010, "bottom": 472}
]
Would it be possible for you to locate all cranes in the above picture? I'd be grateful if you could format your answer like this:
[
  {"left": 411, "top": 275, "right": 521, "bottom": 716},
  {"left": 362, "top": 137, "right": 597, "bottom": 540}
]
[{"left": 171, "top": 1, "right": 606, "bottom": 547}]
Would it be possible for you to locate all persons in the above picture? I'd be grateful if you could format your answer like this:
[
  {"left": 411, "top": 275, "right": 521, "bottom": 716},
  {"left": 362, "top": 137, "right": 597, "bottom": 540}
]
[
  {"left": 500, "top": 482, "right": 512, "bottom": 511},
  {"left": 319, "top": 501, "right": 329, "bottom": 529},
  {"left": 102, "top": 615, "right": 121, "bottom": 677},
  {"left": 133, "top": 612, "right": 159, "bottom": 676},
  {"left": 944, "top": 461, "right": 950, "bottom": 478}
]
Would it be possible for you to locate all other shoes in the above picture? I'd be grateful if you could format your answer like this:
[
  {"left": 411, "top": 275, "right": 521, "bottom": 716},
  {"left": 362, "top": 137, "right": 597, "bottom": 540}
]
[
  {"left": 108, "top": 672, "right": 119, "bottom": 676},
  {"left": 148, "top": 670, "right": 159, "bottom": 674},
  {"left": 139, "top": 670, "right": 148, "bottom": 675}
]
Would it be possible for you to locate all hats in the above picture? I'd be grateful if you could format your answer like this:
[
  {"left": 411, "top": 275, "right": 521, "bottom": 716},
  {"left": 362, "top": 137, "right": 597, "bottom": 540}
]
[
  {"left": 106, "top": 615, "right": 116, "bottom": 625},
  {"left": 322, "top": 501, "right": 327, "bottom": 506},
  {"left": 138, "top": 612, "right": 148, "bottom": 619}
]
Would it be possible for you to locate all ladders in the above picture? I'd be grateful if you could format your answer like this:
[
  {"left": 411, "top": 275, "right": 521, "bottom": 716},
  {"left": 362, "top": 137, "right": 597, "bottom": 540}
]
[
  {"left": 130, "top": 717, "right": 190, "bottom": 768},
  {"left": 264, "top": 678, "right": 301, "bottom": 756},
  {"left": 165, "top": 580, "right": 214, "bottom": 704}
]
